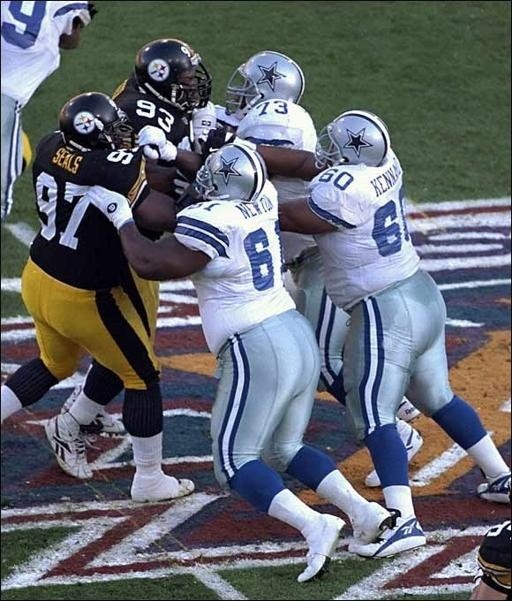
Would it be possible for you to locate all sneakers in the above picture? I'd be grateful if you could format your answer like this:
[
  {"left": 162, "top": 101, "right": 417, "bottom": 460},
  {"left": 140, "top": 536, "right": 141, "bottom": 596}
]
[
  {"left": 130, "top": 471, "right": 195, "bottom": 502},
  {"left": 363, "top": 419, "right": 424, "bottom": 487},
  {"left": 347, "top": 501, "right": 392, "bottom": 555},
  {"left": 60, "top": 385, "right": 128, "bottom": 440},
  {"left": 477, "top": 469, "right": 511, "bottom": 505},
  {"left": 357, "top": 515, "right": 427, "bottom": 559},
  {"left": 396, "top": 396, "right": 423, "bottom": 425},
  {"left": 297, "top": 513, "right": 346, "bottom": 584},
  {"left": 44, "top": 414, "right": 94, "bottom": 480}
]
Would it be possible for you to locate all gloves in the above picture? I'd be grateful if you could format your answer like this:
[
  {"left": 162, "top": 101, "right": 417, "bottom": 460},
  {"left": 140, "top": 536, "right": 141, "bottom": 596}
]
[
  {"left": 198, "top": 126, "right": 236, "bottom": 159},
  {"left": 86, "top": 185, "right": 136, "bottom": 236},
  {"left": 137, "top": 125, "right": 178, "bottom": 163},
  {"left": 174, "top": 169, "right": 191, "bottom": 196},
  {"left": 198, "top": 128, "right": 257, "bottom": 156}
]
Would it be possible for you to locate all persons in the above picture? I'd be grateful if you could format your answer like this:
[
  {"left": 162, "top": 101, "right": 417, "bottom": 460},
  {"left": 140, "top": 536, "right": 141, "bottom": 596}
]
[
  {"left": 0, "top": 88, "right": 196, "bottom": 507},
  {"left": 0, "top": 0, "right": 101, "bottom": 225},
  {"left": 252, "top": 106, "right": 511, "bottom": 562},
  {"left": 229, "top": 96, "right": 426, "bottom": 486},
  {"left": 180, "top": 50, "right": 307, "bottom": 156},
  {"left": 85, "top": 140, "right": 403, "bottom": 586},
  {"left": 464, "top": 516, "right": 511, "bottom": 599},
  {"left": 41, "top": 34, "right": 218, "bottom": 483}
]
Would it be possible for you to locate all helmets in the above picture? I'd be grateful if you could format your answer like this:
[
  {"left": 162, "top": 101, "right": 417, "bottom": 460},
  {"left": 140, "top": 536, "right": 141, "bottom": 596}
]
[
  {"left": 192, "top": 143, "right": 266, "bottom": 202},
  {"left": 314, "top": 110, "right": 390, "bottom": 171},
  {"left": 59, "top": 92, "right": 135, "bottom": 153},
  {"left": 225, "top": 51, "right": 306, "bottom": 120},
  {"left": 134, "top": 39, "right": 212, "bottom": 112}
]
